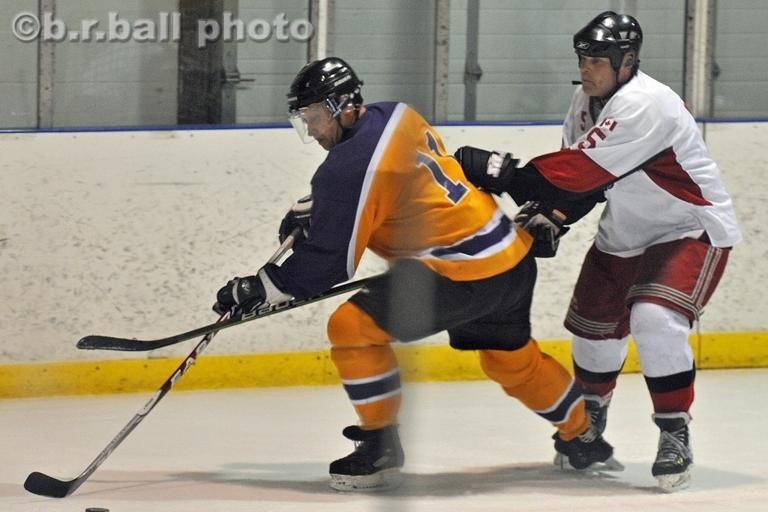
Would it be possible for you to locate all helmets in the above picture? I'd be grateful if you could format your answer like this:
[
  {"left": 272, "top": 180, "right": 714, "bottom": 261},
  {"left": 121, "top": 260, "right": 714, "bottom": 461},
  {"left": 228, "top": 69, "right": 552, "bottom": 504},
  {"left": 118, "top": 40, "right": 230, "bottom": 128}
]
[
  {"left": 288, "top": 57, "right": 363, "bottom": 142},
  {"left": 574, "top": 11, "right": 642, "bottom": 70}
]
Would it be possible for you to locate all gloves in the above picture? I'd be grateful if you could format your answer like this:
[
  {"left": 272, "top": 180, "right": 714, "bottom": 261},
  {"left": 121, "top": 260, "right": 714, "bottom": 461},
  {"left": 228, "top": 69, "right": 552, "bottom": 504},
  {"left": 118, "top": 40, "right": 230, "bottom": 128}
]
[
  {"left": 454, "top": 146, "right": 519, "bottom": 197},
  {"left": 213, "top": 263, "right": 293, "bottom": 317},
  {"left": 279, "top": 194, "right": 313, "bottom": 244},
  {"left": 515, "top": 201, "right": 569, "bottom": 257}
]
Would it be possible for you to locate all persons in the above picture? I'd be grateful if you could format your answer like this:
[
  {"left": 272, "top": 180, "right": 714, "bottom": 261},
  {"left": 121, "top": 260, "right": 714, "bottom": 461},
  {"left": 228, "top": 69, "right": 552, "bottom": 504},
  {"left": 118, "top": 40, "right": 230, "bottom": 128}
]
[
  {"left": 214, "top": 56, "right": 612, "bottom": 476},
  {"left": 455, "top": 11, "right": 739, "bottom": 476}
]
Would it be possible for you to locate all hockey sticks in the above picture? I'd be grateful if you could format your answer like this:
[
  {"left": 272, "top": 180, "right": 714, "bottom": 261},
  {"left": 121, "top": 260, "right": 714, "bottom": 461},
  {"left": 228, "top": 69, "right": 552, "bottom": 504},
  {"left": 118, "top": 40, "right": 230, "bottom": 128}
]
[
  {"left": 24, "top": 227, "right": 301, "bottom": 499},
  {"left": 77, "top": 274, "right": 383, "bottom": 350}
]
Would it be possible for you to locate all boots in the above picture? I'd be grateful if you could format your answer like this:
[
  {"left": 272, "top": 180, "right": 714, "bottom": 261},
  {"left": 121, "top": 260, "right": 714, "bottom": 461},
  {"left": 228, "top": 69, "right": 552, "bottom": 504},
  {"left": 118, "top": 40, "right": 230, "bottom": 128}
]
[
  {"left": 329, "top": 426, "right": 403, "bottom": 475},
  {"left": 552, "top": 389, "right": 613, "bottom": 469},
  {"left": 652, "top": 412, "right": 693, "bottom": 475}
]
